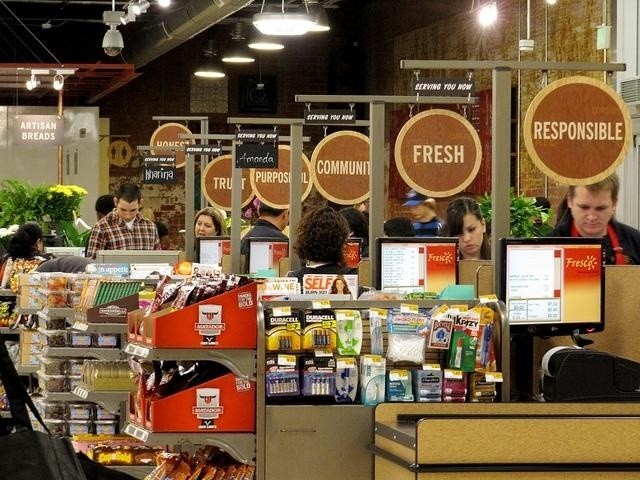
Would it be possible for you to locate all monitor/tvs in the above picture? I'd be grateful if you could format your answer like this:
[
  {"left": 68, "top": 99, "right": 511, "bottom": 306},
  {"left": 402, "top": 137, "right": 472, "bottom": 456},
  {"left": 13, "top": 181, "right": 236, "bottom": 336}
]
[
  {"left": 338, "top": 237, "right": 361, "bottom": 267},
  {"left": 244, "top": 236, "right": 288, "bottom": 278},
  {"left": 499, "top": 236, "right": 606, "bottom": 336},
  {"left": 196, "top": 235, "right": 231, "bottom": 266},
  {"left": 375, "top": 236, "right": 460, "bottom": 296}
]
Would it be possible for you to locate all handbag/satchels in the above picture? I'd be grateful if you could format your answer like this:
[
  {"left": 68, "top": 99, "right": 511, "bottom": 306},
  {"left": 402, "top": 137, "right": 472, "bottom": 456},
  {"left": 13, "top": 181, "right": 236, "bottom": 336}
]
[{"left": 0, "top": 431, "right": 87, "bottom": 480}]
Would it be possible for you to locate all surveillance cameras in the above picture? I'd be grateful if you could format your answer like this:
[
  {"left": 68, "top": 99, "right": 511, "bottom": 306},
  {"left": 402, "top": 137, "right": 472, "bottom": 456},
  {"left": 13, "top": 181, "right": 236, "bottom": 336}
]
[{"left": 102, "top": 25, "right": 125, "bottom": 56}]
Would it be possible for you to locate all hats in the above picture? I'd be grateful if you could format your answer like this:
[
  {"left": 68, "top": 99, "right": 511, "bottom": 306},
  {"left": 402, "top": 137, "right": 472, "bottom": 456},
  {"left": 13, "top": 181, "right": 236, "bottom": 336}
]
[{"left": 401, "top": 189, "right": 426, "bottom": 206}]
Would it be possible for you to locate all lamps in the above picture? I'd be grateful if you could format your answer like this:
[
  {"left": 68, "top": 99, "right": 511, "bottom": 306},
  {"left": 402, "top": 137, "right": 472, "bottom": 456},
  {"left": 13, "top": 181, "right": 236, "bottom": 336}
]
[
  {"left": 26, "top": 75, "right": 41, "bottom": 90},
  {"left": 248, "top": 33, "right": 285, "bottom": 51},
  {"left": 295, "top": 4, "right": 331, "bottom": 32},
  {"left": 221, "top": 38, "right": 256, "bottom": 64},
  {"left": 193, "top": 39, "right": 226, "bottom": 79},
  {"left": 53, "top": 75, "right": 64, "bottom": 90},
  {"left": 132, "top": 1, "right": 150, "bottom": 15},
  {"left": 120, "top": 13, "right": 136, "bottom": 26}
]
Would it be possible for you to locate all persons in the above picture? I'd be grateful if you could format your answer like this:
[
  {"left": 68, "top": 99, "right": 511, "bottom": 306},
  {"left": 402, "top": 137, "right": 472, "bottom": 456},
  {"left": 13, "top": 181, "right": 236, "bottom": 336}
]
[
  {"left": 531, "top": 196, "right": 554, "bottom": 238},
  {"left": 541, "top": 171, "right": 640, "bottom": 265},
  {"left": 84, "top": 194, "right": 116, "bottom": 257},
  {"left": 285, "top": 185, "right": 448, "bottom": 299},
  {"left": 329, "top": 277, "right": 351, "bottom": 295},
  {"left": 86, "top": 182, "right": 162, "bottom": 259},
  {"left": 192, "top": 206, "right": 229, "bottom": 237},
  {"left": 241, "top": 201, "right": 289, "bottom": 274},
  {"left": 436, "top": 196, "right": 492, "bottom": 261},
  {"left": 0, "top": 220, "right": 57, "bottom": 260}
]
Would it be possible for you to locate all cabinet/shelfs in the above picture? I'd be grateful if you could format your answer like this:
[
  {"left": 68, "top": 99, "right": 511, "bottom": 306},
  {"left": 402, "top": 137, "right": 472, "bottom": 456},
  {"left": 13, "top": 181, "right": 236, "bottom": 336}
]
[
  {"left": 124, "top": 297, "right": 512, "bottom": 479},
  {"left": 33, "top": 269, "right": 138, "bottom": 439}
]
